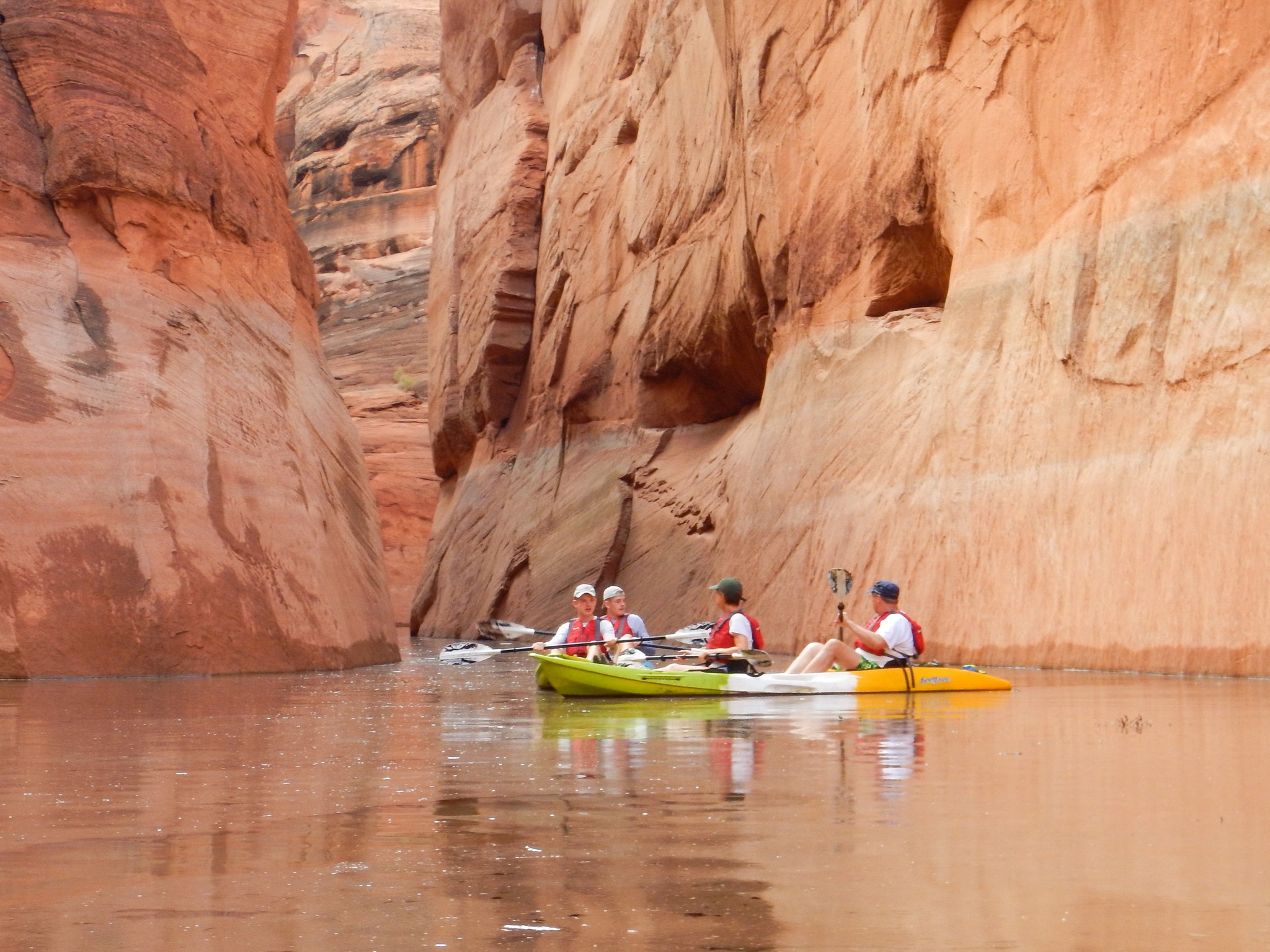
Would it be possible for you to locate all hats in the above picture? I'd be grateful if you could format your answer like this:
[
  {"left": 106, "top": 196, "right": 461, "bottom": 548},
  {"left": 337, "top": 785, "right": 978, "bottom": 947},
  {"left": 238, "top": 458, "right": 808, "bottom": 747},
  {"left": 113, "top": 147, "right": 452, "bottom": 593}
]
[
  {"left": 574, "top": 584, "right": 597, "bottom": 598},
  {"left": 864, "top": 581, "right": 900, "bottom": 600},
  {"left": 708, "top": 578, "right": 748, "bottom": 602},
  {"left": 601, "top": 586, "right": 624, "bottom": 609}
]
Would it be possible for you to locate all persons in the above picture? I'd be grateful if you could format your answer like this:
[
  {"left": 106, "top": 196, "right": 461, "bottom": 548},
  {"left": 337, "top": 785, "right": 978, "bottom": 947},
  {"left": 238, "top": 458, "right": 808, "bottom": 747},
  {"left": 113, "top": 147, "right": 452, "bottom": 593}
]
[
  {"left": 653, "top": 576, "right": 753, "bottom": 673},
  {"left": 598, "top": 585, "right": 655, "bottom": 670},
  {"left": 532, "top": 584, "right": 618, "bottom": 663},
  {"left": 784, "top": 581, "right": 915, "bottom": 674}
]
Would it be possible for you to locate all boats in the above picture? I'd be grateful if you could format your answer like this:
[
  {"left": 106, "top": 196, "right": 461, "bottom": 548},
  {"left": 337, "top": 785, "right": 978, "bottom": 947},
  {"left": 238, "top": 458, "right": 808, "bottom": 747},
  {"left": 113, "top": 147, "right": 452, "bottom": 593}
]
[
  {"left": 533, "top": 653, "right": 621, "bottom": 691},
  {"left": 528, "top": 650, "right": 1014, "bottom": 700}
]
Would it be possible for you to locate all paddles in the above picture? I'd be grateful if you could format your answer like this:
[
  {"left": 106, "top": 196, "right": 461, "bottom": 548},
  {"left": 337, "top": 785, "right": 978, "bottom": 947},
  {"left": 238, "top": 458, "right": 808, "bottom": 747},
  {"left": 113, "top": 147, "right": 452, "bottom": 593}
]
[
  {"left": 837, "top": 602, "right": 845, "bottom": 642},
  {"left": 439, "top": 621, "right": 715, "bottom": 665},
  {"left": 617, "top": 649, "right": 772, "bottom": 667},
  {"left": 496, "top": 620, "right": 706, "bottom": 652}
]
